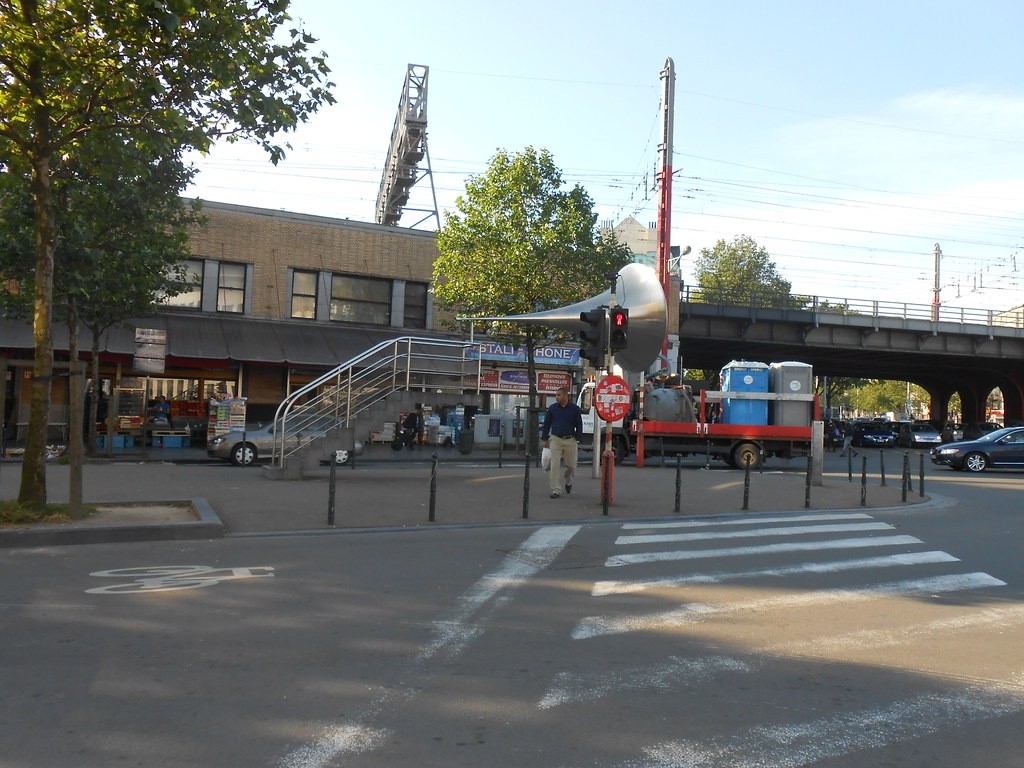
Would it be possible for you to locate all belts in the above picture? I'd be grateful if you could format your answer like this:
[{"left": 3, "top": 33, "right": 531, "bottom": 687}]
[{"left": 551, "top": 431, "right": 575, "bottom": 440}]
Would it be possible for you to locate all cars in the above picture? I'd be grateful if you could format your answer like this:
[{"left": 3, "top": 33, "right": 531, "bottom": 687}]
[
  {"left": 823, "top": 419, "right": 852, "bottom": 447},
  {"left": 942, "top": 422, "right": 964, "bottom": 442},
  {"left": 978, "top": 421, "right": 1004, "bottom": 438},
  {"left": 899, "top": 422, "right": 943, "bottom": 449},
  {"left": 929, "top": 426, "right": 1024, "bottom": 473},
  {"left": 205, "top": 410, "right": 365, "bottom": 467},
  {"left": 849, "top": 421, "right": 896, "bottom": 449}
]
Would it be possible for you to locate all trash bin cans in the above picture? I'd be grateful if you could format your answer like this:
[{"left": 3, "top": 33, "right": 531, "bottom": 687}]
[
  {"left": 719, "top": 359, "right": 813, "bottom": 427},
  {"left": 455, "top": 429, "right": 474, "bottom": 455}
]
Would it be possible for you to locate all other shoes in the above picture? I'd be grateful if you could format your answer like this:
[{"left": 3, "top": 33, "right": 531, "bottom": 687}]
[{"left": 550, "top": 482, "right": 573, "bottom": 499}]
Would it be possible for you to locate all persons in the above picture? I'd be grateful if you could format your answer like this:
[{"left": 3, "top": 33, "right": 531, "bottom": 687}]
[
  {"left": 840, "top": 418, "right": 859, "bottom": 458},
  {"left": 151, "top": 396, "right": 175, "bottom": 432},
  {"left": 401, "top": 407, "right": 422, "bottom": 450},
  {"left": 710, "top": 411, "right": 721, "bottom": 460},
  {"left": 540, "top": 388, "right": 584, "bottom": 498},
  {"left": 824, "top": 418, "right": 839, "bottom": 453}
]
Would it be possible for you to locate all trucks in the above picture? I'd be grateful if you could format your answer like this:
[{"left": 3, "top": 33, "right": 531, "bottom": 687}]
[{"left": 574, "top": 381, "right": 820, "bottom": 475}]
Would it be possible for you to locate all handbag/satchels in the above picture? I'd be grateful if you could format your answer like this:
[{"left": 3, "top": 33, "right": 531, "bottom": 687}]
[{"left": 541, "top": 446, "right": 551, "bottom": 472}]
